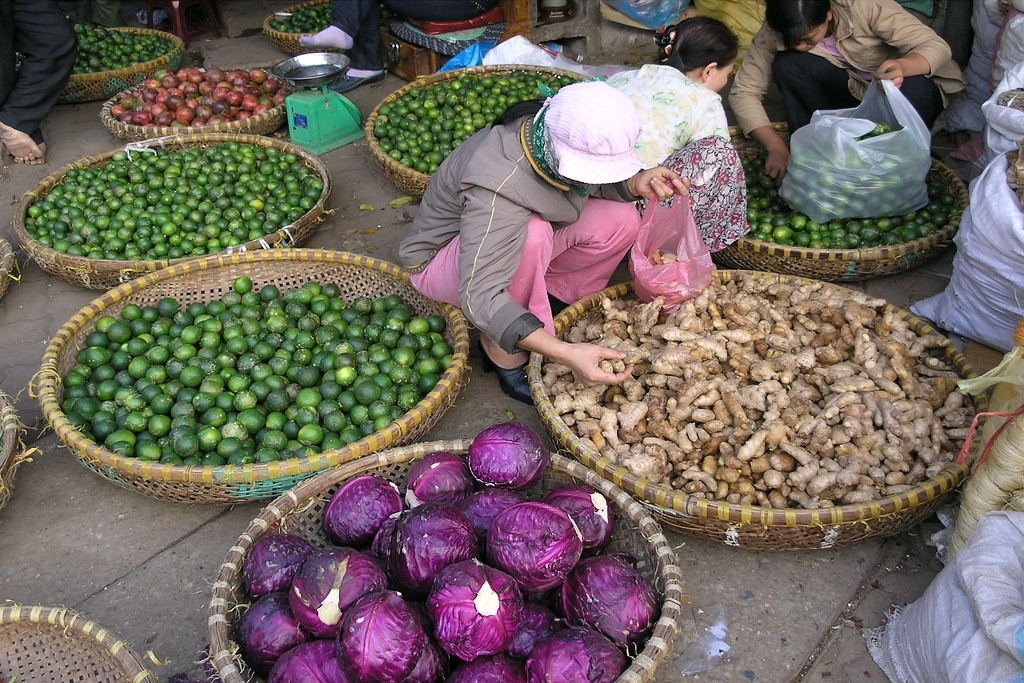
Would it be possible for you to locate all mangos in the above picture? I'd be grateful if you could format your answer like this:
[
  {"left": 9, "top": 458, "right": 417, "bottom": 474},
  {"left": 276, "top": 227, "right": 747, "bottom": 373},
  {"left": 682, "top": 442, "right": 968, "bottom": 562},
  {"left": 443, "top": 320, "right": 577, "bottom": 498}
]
[{"left": 109, "top": 67, "right": 291, "bottom": 127}]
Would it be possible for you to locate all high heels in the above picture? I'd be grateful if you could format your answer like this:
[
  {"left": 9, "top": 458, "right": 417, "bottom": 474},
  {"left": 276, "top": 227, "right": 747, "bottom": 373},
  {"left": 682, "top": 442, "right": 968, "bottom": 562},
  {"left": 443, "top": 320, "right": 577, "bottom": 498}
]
[
  {"left": 476, "top": 338, "right": 535, "bottom": 406},
  {"left": 548, "top": 293, "right": 569, "bottom": 315}
]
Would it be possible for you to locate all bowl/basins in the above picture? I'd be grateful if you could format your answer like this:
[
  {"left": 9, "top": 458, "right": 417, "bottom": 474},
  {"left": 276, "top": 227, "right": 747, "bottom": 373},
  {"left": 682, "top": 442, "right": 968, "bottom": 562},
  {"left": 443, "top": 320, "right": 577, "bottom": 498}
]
[{"left": 273, "top": 13, "right": 293, "bottom": 23}]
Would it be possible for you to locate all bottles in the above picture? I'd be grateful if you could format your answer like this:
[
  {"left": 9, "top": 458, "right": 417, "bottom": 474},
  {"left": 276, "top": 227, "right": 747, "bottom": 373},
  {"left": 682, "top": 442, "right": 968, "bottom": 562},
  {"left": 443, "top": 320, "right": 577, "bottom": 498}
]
[{"left": 546, "top": 41, "right": 584, "bottom": 63}]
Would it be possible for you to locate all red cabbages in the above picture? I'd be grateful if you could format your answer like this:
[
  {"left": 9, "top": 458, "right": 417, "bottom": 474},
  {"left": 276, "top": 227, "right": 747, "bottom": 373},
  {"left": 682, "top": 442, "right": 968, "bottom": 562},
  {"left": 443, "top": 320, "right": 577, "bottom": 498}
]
[{"left": 240, "top": 422, "right": 656, "bottom": 683}]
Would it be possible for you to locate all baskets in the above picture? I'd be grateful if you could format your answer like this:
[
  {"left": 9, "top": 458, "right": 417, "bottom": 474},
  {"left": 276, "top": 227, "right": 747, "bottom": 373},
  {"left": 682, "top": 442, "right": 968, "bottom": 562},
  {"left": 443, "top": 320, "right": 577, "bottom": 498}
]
[{"left": 0, "top": 0, "right": 1024, "bottom": 683}]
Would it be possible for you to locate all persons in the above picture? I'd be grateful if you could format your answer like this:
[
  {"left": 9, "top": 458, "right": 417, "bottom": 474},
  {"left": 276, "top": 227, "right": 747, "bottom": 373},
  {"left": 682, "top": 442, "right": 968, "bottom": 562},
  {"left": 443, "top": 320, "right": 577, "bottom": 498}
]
[
  {"left": 397, "top": 81, "right": 690, "bottom": 405},
  {"left": 727, "top": 0, "right": 966, "bottom": 181},
  {"left": 611, "top": 16, "right": 748, "bottom": 254},
  {"left": 0, "top": 0, "right": 79, "bottom": 165},
  {"left": 299, "top": 0, "right": 504, "bottom": 93},
  {"left": 657, "top": 0, "right": 768, "bottom": 75}
]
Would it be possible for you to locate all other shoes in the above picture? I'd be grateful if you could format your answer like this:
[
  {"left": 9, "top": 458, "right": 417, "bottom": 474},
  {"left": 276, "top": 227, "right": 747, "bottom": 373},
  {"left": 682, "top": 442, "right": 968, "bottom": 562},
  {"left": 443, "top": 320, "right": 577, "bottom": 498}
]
[{"left": 328, "top": 71, "right": 385, "bottom": 93}]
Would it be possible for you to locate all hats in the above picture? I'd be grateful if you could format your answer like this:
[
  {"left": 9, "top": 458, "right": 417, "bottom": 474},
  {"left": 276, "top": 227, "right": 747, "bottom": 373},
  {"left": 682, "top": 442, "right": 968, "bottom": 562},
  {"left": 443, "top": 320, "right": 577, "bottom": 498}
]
[{"left": 534, "top": 81, "right": 642, "bottom": 185}]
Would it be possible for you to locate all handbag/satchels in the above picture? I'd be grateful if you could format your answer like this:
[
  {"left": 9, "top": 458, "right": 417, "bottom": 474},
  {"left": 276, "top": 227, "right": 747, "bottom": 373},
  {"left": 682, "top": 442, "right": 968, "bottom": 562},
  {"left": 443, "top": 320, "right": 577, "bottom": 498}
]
[
  {"left": 629, "top": 181, "right": 710, "bottom": 316},
  {"left": 779, "top": 76, "right": 931, "bottom": 225},
  {"left": 603, "top": 0, "right": 690, "bottom": 31}
]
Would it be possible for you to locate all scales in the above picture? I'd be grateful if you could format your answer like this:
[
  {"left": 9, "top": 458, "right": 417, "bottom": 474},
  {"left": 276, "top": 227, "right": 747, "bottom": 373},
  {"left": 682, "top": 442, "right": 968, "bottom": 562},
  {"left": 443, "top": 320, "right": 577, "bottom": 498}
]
[{"left": 271, "top": 52, "right": 366, "bottom": 157}]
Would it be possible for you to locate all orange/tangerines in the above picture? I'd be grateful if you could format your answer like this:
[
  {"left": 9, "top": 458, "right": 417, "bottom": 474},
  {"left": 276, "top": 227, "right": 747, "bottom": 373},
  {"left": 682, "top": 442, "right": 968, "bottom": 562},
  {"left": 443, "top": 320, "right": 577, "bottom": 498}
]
[
  {"left": 270, "top": 3, "right": 335, "bottom": 33},
  {"left": 65, "top": 22, "right": 175, "bottom": 104},
  {"left": 25, "top": 142, "right": 323, "bottom": 262},
  {"left": 374, "top": 69, "right": 581, "bottom": 176},
  {"left": 736, "top": 121, "right": 958, "bottom": 249},
  {"left": 61, "top": 278, "right": 453, "bottom": 467}
]
